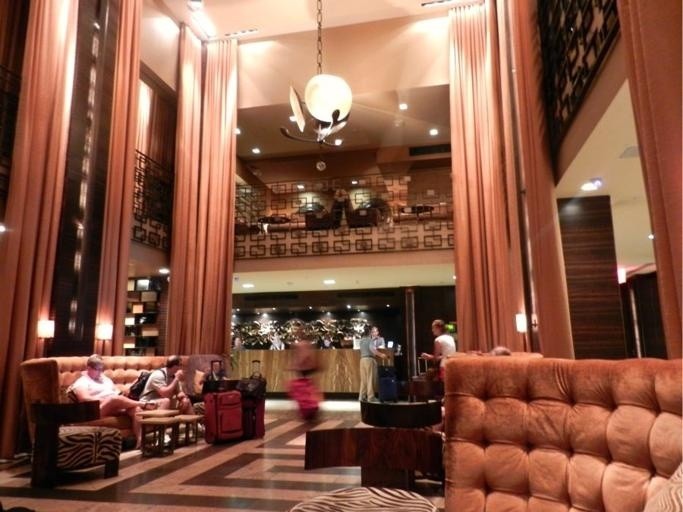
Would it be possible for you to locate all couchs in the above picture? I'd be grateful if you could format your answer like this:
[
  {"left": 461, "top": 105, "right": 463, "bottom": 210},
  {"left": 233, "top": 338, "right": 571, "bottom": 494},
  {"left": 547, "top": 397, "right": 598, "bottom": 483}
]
[
  {"left": 22, "top": 354, "right": 269, "bottom": 486},
  {"left": 441, "top": 356, "right": 680, "bottom": 511}
]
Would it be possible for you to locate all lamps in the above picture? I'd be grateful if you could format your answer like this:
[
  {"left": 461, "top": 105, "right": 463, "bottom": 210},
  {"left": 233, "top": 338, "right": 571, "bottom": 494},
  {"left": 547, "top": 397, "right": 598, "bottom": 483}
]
[
  {"left": 281, "top": 1, "right": 357, "bottom": 148},
  {"left": 94, "top": 323, "right": 113, "bottom": 341},
  {"left": 35, "top": 321, "right": 53, "bottom": 341}
]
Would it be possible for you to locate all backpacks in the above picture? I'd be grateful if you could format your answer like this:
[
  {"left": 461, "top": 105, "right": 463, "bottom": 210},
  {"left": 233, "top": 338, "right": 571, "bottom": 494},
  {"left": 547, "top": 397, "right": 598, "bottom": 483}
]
[{"left": 128, "top": 372, "right": 152, "bottom": 401}]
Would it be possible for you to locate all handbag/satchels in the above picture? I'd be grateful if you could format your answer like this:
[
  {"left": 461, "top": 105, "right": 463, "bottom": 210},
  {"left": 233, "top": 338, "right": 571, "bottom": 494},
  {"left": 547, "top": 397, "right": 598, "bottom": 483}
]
[
  {"left": 236, "top": 372, "right": 268, "bottom": 397},
  {"left": 376, "top": 366, "right": 398, "bottom": 377}
]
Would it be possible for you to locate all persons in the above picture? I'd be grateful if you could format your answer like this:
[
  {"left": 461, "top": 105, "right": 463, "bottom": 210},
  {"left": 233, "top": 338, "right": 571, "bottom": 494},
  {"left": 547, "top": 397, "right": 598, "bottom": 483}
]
[
  {"left": 370, "top": 326, "right": 384, "bottom": 350},
  {"left": 320, "top": 333, "right": 335, "bottom": 350},
  {"left": 267, "top": 333, "right": 284, "bottom": 350},
  {"left": 71, "top": 354, "right": 157, "bottom": 449},
  {"left": 139, "top": 355, "right": 205, "bottom": 445},
  {"left": 357, "top": 325, "right": 385, "bottom": 403},
  {"left": 231, "top": 324, "right": 244, "bottom": 349},
  {"left": 420, "top": 319, "right": 456, "bottom": 433},
  {"left": 204, "top": 360, "right": 241, "bottom": 382},
  {"left": 176, "top": 355, "right": 204, "bottom": 405}
]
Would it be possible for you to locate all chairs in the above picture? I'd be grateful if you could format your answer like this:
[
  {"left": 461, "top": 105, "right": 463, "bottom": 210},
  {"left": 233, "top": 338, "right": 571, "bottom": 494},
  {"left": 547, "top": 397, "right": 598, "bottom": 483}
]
[{"left": 304, "top": 189, "right": 379, "bottom": 231}]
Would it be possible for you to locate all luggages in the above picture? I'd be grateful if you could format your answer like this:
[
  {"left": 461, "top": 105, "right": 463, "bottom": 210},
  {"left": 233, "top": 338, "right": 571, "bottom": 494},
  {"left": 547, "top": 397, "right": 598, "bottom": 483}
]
[
  {"left": 203, "top": 357, "right": 238, "bottom": 397},
  {"left": 205, "top": 390, "right": 245, "bottom": 444},
  {"left": 407, "top": 356, "right": 433, "bottom": 402},
  {"left": 377, "top": 377, "right": 399, "bottom": 403},
  {"left": 247, "top": 360, "right": 265, "bottom": 439}
]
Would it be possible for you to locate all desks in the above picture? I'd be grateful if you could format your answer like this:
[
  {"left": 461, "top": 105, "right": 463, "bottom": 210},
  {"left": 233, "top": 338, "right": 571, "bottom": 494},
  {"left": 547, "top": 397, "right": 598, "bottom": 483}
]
[
  {"left": 397, "top": 205, "right": 435, "bottom": 224},
  {"left": 257, "top": 216, "right": 297, "bottom": 234}
]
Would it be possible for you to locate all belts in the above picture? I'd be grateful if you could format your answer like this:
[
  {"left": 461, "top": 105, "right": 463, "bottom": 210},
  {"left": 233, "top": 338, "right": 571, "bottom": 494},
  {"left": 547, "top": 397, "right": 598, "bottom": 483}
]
[{"left": 362, "top": 355, "right": 374, "bottom": 358}]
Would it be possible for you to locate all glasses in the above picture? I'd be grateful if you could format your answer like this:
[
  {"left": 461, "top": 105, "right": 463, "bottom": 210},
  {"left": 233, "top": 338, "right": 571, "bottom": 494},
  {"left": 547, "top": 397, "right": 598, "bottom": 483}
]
[{"left": 89, "top": 366, "right": 105, "bottom": 371}]
